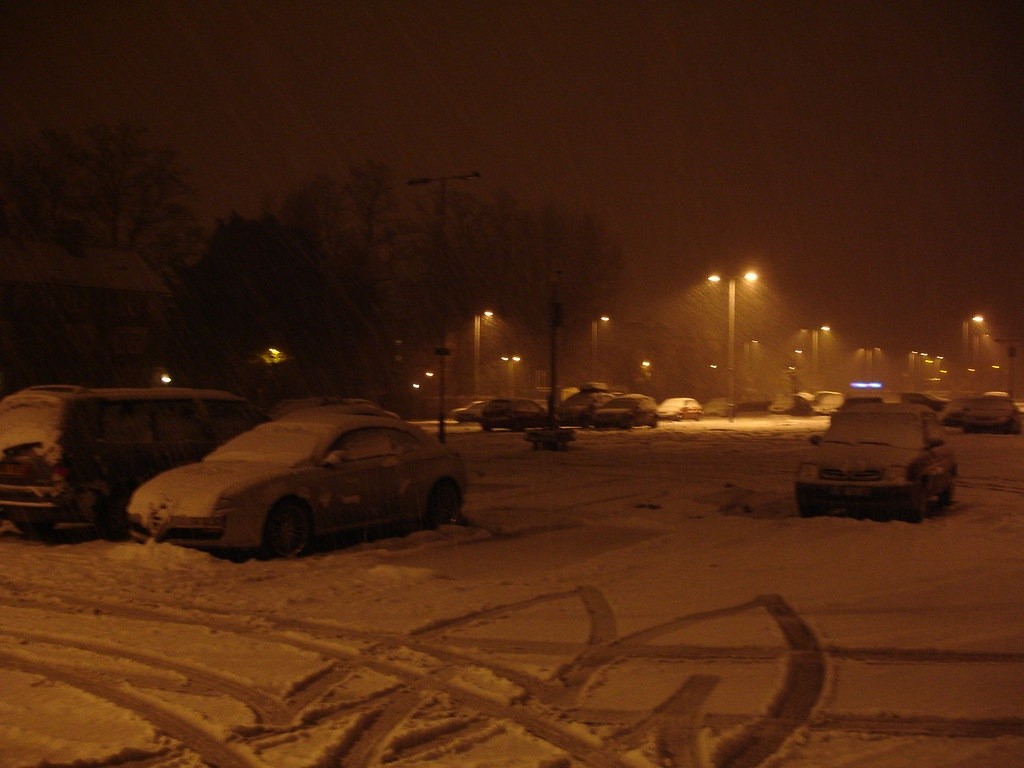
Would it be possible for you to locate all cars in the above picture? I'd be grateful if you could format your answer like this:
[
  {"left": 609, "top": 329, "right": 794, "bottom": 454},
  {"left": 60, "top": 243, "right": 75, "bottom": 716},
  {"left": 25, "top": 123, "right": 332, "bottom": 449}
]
[
  {"left": 766, "top": 390, "right": 847, "bottom": 415},
  {"left": 795, "top": 400, "right": 959, "bottom": 523},
  {"left": 592, "top": 394, "right": 659, "bottom": 429},
  {"left": 656, "top": 398, "right": 704, "bottom": 422},
  {"left": 897, "top": 392, "right": 959, "bottom": 427},
  {"left": 451, "top": 396, "right": 560, "bottom": 431},
  {"left": 960, "top": 389, "right": 1017, "bottom": 435},
  {"left": 125, "top": 409, "right": 471, "bottom": 562},
  {"left": 273, "top": 396, "right": 401, "bottom": 422}
]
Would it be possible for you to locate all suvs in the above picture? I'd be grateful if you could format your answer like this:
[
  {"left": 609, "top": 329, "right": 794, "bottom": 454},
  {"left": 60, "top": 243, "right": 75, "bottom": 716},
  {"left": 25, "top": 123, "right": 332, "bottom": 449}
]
[
  {"left": 0, "top": 383, "right": 272, "bottom": 543},
  {"left": 555, "top": 381, "right": 625, "bottom": 428}
]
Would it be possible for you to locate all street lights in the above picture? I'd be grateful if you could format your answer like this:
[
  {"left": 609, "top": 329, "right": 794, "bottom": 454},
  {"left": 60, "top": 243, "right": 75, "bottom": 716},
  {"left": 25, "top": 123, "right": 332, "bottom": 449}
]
[
  {"left": 706, "top": 267, "right": 757, "bottom": 424},
  {"left": 407, "top": 171, "right": 484, "bottom": 443}
]
